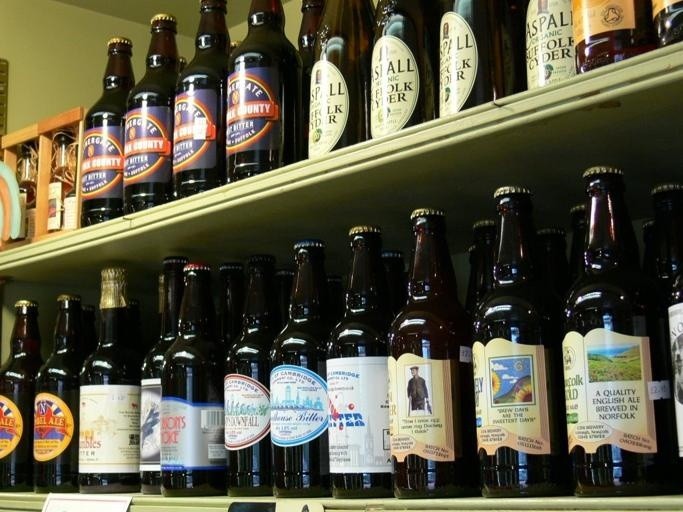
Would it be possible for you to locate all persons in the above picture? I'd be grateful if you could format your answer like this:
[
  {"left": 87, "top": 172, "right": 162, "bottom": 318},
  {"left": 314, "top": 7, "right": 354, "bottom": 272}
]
[{"left": 406, "top": 366, "right": 428, "bottom": 411}]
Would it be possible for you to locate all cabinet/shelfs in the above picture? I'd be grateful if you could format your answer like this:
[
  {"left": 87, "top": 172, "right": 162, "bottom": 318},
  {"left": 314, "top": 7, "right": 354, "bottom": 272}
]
[{"left": 0, "top": 42, "right": 681, "bottom": 510}]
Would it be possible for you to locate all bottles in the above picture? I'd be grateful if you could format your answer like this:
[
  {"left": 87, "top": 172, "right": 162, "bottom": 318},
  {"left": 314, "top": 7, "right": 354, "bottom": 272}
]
[{"left": 0, "top": 1, "right": 683, "bottom": 496}]
